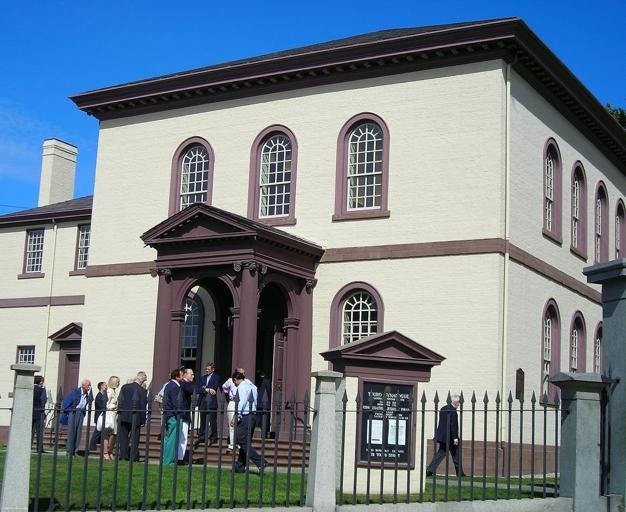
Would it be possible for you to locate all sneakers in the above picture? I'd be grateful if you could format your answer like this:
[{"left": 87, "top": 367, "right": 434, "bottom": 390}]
[
  {"left": 456, "top": 474, "right": 466, "bottom": 477},
  {"left": 427, "top": 470, "right": 434, "bottom": 476}
]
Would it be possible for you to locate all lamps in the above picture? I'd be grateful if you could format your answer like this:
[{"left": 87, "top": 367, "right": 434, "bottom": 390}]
[
  {"left": 234, "top": 260, "right": 267, "bottom": 274},
  {"left": 150, "top": 267, "right": 171, "bottom": 280},
  {"left": 306, "top": 277, "right": 317, "bottom": 288}
]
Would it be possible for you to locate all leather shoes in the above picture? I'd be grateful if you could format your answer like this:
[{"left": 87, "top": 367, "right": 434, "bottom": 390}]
[{"left": 36, "top": 449, "right": 268, "bottom": 474}]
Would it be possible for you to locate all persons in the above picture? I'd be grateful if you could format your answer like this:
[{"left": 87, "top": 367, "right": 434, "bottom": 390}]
[
  {"left": 427, "top": 395, "right": 466, "bottom": 477},
  {"left": 31, "top": 361, "right": 272, "bottom": 475}
]
